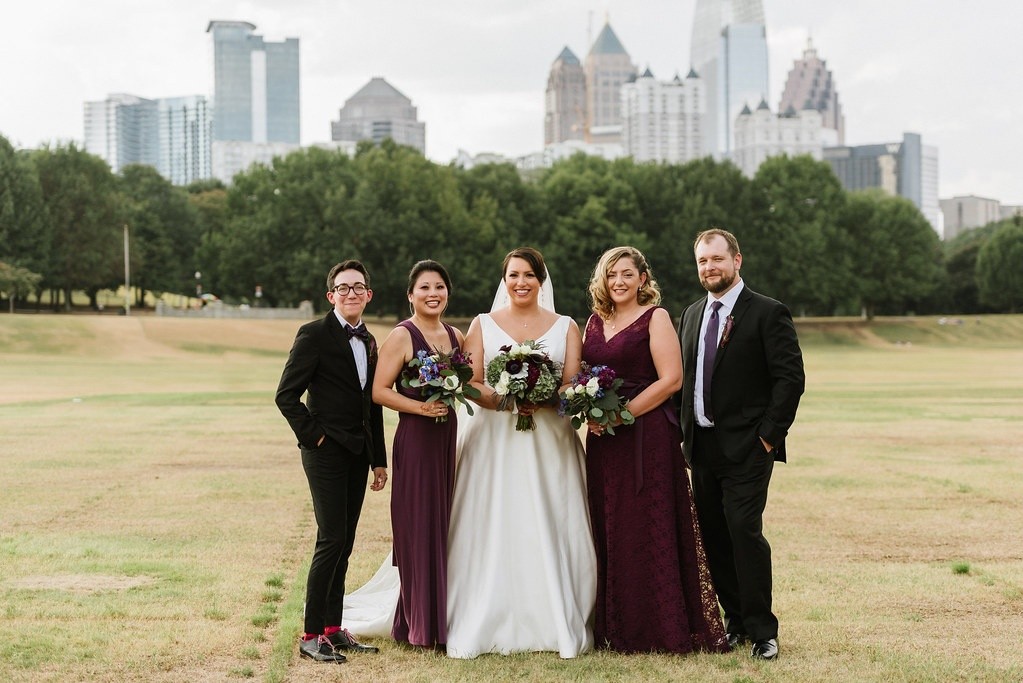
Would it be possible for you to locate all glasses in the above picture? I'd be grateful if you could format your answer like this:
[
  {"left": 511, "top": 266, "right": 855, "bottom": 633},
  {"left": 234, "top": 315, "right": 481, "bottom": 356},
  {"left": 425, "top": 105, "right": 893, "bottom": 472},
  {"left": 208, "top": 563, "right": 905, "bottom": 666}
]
[{"left": 330, "top": 282, "right": 370, "bottom": 296}]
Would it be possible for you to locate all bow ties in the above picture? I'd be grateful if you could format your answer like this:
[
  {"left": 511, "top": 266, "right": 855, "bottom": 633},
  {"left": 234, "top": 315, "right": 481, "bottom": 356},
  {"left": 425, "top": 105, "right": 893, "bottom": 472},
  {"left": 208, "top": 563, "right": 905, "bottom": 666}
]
[{"left": 345, "top": 323, "right": 368, "bottom": 340}]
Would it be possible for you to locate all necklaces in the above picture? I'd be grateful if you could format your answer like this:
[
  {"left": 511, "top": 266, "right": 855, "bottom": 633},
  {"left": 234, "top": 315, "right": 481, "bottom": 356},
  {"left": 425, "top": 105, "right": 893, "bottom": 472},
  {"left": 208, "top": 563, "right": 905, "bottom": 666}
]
[
  {"left": 506, "top": 307, "right": 543, "bottom": 327},
  {"left": 609, "top": 305, "right": 643, "bottom": 330}
]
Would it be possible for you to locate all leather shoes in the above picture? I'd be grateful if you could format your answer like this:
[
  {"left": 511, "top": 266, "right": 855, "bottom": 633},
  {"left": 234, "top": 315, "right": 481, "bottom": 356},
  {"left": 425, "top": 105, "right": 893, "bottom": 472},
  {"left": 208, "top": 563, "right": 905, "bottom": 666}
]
[
  {"left": 299, "top": 634, "right": 347, "bottom": 664},
  {"left": 751, "top": 638, "right": 780, "bottom": 661},
  {"left": 326, "top": 628, "right": 379, "bottom": 654},
  {"left": 717, "top": 632, "right": 745, "bottom": 652}
]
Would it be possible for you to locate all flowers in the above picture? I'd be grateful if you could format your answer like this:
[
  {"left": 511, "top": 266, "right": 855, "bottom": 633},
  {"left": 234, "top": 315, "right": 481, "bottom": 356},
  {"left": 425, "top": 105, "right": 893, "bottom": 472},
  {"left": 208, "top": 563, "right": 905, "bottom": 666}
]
[
  {"left": 401, "top": 342, "right": 482, "bottom": 424},
  {"left": 558, "top": 359, "right": 636, "bottom": 436},
  {"left": 486, "top": 339, "right": 563, "bottom": 432}
]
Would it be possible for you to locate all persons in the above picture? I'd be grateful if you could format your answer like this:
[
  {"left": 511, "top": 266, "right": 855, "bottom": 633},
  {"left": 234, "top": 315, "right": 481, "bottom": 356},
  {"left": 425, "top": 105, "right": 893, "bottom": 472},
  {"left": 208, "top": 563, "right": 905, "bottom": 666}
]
[
  {"left": 371, "top": 259, "right": 465, "bottom": 646},
  {"left": 580, "top": 246, "right": 684, "bottom": 657},
  {"left": 676, "top": 229, "right": 806, "bottom": 660},
  {"left": 275, "top": 259, "right": 388, "bottom": 665},
  {"left": 462, "top": 245, "right": 583, "bottom": 661}
]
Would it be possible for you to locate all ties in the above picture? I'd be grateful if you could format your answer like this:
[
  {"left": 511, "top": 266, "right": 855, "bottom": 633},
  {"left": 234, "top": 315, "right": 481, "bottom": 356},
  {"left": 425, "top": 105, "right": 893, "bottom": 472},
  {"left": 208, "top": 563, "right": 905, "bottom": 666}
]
[{"left": 702, "top": 302, "right": 723, "bottom": 422}]
